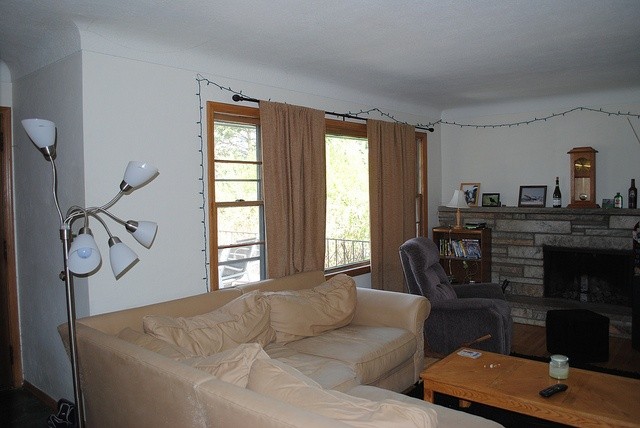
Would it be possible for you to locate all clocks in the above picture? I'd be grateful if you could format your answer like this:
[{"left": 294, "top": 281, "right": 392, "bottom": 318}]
[{"left": 566, "top": 147, "right": 600, "bottom": 209}]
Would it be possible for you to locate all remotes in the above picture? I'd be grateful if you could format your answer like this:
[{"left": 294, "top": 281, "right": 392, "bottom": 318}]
[{"left": 541, "top": 384, "right": 567, "bottom": 397}]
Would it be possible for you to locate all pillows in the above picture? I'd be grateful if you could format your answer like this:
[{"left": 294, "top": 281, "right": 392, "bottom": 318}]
[
  {"left": 143, "top": 289, "right": 276, "bottom": 350},
  {"left": 246, "top": 359, "right": 438, "bottom": 428},
  {"left": 120, "top": 328, "right": 270, "bottom": 388},
  {"left": 262, "top": 274, "right": 358, "bottom": 344}
]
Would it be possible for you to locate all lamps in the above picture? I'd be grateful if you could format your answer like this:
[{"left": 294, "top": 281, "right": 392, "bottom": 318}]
[
  {"left": 446, "top": 190, "right": 470, "bottom": 229},
  {"left": 21, "top": 118, "right": 159, "bottom": 426}
]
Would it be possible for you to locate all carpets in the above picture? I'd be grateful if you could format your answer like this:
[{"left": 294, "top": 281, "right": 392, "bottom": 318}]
[{"left": 406, "top": 350, "right": 640, "bottom": 428}]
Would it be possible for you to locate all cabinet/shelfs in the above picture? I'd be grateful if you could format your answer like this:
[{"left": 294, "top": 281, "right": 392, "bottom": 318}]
[{"left": 432, "top": 225, "right": 491, "bottom": 283}]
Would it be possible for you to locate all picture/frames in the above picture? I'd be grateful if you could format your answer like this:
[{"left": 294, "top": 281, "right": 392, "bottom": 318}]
[
  {"left": 482, "top": 192, "right": 500, "bottom": 207},
  {"left": 518, "top": 185, "right": 548, "bottom": 208},
  {"left": 459, "top": 183, "right": 480, "bottom": 208}
]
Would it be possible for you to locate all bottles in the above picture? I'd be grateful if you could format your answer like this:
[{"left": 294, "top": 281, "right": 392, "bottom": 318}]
[
  {"left": 549, "top": 356, "right": 569, "bottom": 379},
  {"left": 552, "top": 176, "right": 562, "bottom": 207},
  {"left": 614, "top": 192, "right": 623, "bottom": 209},
  {"left": 628, "top": 179, "right": 637, "bottom": 209}
]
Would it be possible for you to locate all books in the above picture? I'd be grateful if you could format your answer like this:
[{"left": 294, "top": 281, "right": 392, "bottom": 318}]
[{"left": 439, "top": 239, "right": 480, "bottom": 258}]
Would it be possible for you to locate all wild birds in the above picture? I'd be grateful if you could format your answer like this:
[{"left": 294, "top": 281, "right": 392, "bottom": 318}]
[{"left": 490, "top": 197, "right": 497, "bottom": 205}]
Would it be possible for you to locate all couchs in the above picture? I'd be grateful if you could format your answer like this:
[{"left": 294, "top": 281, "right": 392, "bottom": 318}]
[
  {"left": 399, "top": 238, "right": 513, "bottom": 356},
  {"left": 57, "top": 271, "right": 503, "bottom": 428}
]
